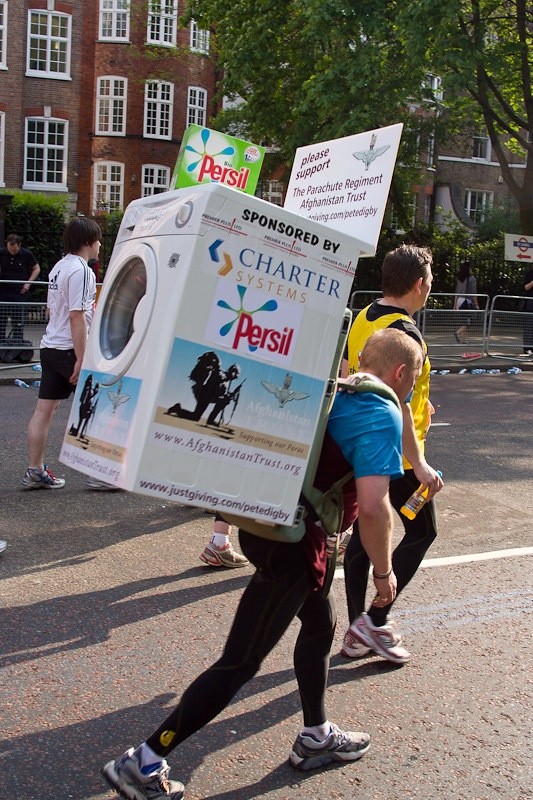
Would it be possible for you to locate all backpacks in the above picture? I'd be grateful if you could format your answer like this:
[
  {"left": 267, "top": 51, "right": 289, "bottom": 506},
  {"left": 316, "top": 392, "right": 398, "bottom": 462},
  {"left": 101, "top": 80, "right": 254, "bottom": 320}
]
[
  {"left": 188, "top": 350, "right": 220, "bottom": 383},
  {"left": 80, "top": 374, "right": 93, "bottom": 404}
]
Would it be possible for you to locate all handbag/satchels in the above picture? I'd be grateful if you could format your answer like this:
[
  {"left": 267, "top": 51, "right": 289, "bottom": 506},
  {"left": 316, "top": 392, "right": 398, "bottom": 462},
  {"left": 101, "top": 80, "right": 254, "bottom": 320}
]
[{"left": 0, "top": 328, "right": 35, "bottom": 364}]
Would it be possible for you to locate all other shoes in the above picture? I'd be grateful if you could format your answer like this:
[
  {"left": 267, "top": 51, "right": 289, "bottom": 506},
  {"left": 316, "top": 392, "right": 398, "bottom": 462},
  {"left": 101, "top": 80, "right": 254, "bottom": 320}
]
[
  {"left": 199, "top": 542, "right": 249, "bottom": 568},
  {"left": 461, "top": 342, "right": 469, "bottom": 344},
  {"left": 0, "top": 540, "right": 8, "bottom": 552},
  {"left": 167, "top": 403, "right": 180, "bottom": 414},
  {"left": 453, "top": 332, "right": 460, "bottom": 344},
  {"left": 84, "top": 475, "right": 121, "bottom": 490},
  {"left": 524, "top": 350, "right": 533, "bottom": 354},
  {"left": 326, "top": 531, "right": 352, "bottom": 560}
]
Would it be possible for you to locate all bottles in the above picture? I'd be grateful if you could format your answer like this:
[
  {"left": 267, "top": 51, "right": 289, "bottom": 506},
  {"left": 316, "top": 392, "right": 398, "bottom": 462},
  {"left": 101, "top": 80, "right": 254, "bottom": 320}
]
[
  {"left": 15, "top": 379, "right": 29, "bottom": 388},
  {"left": 487, "top": 368, "right": 500, "bottom": 373},
  {"left": 458, "top": 369, "right": 467, "bottom": 374},
  {"left": 470, "top": 369, "right": 486, "bottom": 374},
  {"left": 507, "top": 367, "right": 522, "bottom": 374},
  {"left": 430, "top": 370, "right": 451, "bottom": 375},
  {"left": 32, "top": 381, "right": 41, "bottom": 387},
  {"left": 32, "top": 364, "right": 42, "bottom": 371},
  {"left": 401, "top": 469, "right": 443, "bottom": 520}
]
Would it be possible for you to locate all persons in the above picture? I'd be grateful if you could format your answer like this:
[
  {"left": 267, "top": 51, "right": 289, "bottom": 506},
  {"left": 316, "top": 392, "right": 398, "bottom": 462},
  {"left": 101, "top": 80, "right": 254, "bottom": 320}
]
[
  {"left": 19, "top": 218, "right": 120, "bottom": 489},
  {"left": 0, "top": 234, "right": 41, "bottom": 339},
  {"left": 99, "top": 328, "right": 424, "bottom": 800},
  {"left": 181, "top": 244, "right": 533, "bottom": 664}
]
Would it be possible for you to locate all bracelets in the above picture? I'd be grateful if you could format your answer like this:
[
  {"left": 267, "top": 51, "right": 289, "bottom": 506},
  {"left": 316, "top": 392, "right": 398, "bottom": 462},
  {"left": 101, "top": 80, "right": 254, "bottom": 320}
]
[{"left": 373, "top": 567, "right": 393, "bottom": 579}]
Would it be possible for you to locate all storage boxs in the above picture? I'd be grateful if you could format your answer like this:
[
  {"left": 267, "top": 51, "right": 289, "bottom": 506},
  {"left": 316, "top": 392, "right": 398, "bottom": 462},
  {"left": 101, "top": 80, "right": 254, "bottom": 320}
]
[{"left": 168, "top": 123, "right": 266, "bottom": 195}]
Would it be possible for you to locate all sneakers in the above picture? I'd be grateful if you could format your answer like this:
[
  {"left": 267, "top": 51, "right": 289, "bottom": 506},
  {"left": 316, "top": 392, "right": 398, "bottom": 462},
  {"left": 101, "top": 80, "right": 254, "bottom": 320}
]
[
  {"left": 20, "top": 463, "right": 65, "bottom": 489},
  {"left": 339, "top": 630, "right": 379, "bottom": 658},
  {"left": 350, "top": 612, "right": 412, "bottom": 664},
  {"left": 100, "top": 747, "right": 185, "bottom": 800},
  {"left": 288, "top": 722, "right": 371, "bottom": 770}
]
